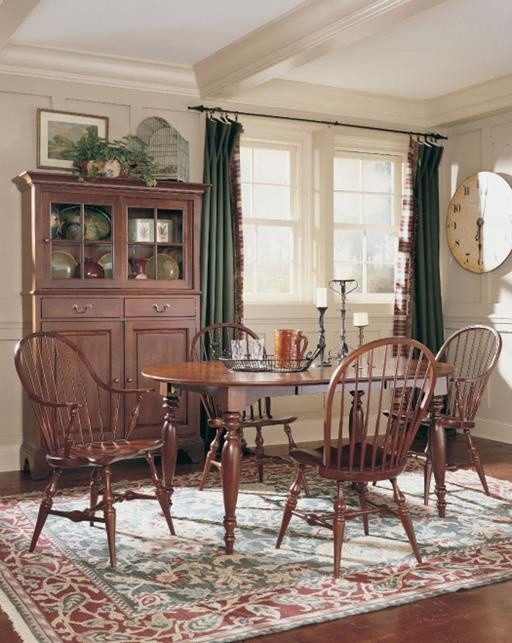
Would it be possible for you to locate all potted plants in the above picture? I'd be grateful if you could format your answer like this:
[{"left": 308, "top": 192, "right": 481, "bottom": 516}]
[{"left": 70, "top": 124, "right": 159, "bottom": 187}]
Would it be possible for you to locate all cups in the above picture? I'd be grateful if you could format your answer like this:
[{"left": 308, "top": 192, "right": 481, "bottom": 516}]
[
  {"left": 273, "top": 327, "right": 309, "bottom": 370},
  {"left": 231, "top": 339, "right": 264, "bottom": 362}
]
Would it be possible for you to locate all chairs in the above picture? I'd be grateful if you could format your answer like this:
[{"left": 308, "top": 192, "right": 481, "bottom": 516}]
[
  {"left": 191, "top": 322, "right": 309, "bottom": 496},
  {"left": 274, "top": 335, "right": 438, "bottom": 579},
  {"left": 14, "top": 328, "right": 176, "bottom": 570},
  {"left": 381, "top": 324, "right": 502, "bottom": 520}
]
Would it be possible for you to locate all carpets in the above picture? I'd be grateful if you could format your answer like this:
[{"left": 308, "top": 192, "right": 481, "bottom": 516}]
[{"left": 0, "top": 450, "right": 512, "bottom": 643}]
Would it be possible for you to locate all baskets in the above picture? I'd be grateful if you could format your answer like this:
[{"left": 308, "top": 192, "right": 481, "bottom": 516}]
[{"left": 218, "top": 353, "right": 313, "bottom": 374}]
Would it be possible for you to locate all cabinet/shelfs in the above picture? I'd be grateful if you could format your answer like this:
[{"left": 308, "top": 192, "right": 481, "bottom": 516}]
[{"left": 10, "top": 169, "right": 206, "bottom": 482}]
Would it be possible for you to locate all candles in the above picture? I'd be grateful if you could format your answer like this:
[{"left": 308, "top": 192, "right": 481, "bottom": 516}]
[
  {"left": 353, "top": 312, "right": 370, "bottom": 326},
  {"left": 317, "top": 288, "right": 329, "bottom": 309}
]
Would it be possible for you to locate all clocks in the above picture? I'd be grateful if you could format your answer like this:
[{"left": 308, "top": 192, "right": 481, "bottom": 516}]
[{"left": 446, "top": 172, "right": 512, "bottom": 274}]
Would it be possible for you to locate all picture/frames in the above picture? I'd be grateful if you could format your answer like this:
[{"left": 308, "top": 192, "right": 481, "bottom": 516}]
[{"left": 37, "top": 107, "right": 109, "bottom": 171}]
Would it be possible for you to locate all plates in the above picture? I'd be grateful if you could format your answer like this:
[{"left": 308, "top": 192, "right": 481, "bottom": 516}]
[{"left": 51, "top": 203, "right": 183, "bottom": 280}]
